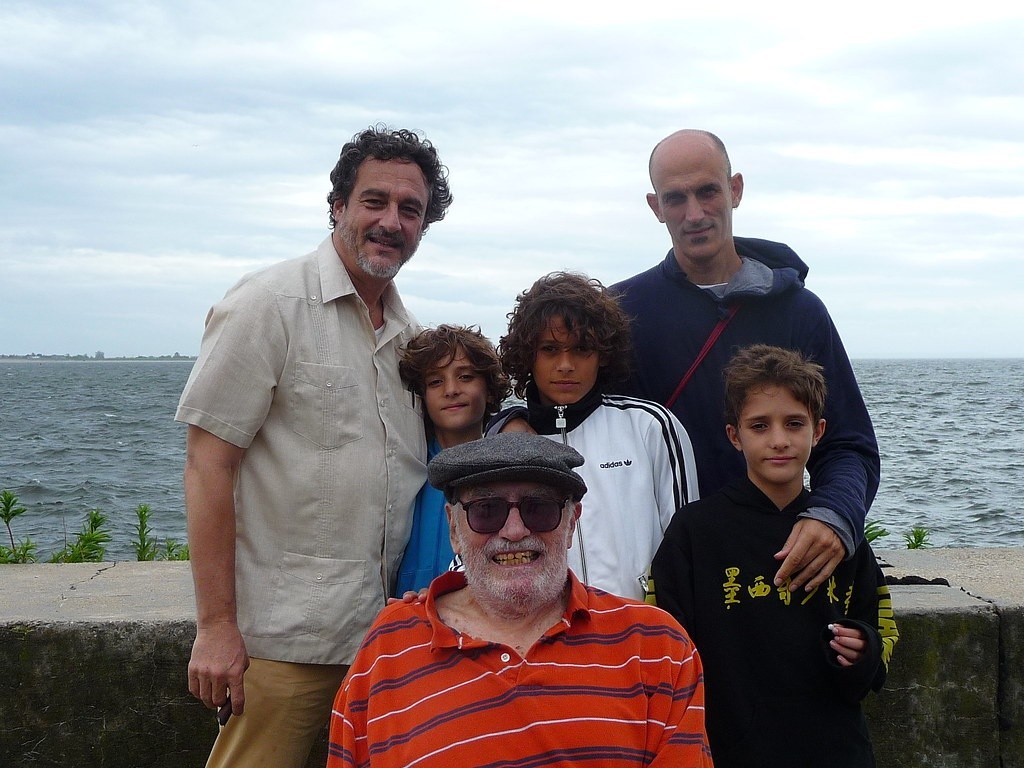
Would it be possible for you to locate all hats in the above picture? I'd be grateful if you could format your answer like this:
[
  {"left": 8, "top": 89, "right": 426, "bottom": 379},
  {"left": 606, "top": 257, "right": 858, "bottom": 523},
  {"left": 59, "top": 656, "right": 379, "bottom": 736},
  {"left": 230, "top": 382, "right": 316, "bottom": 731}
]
[{"left": 428, "top": 431, "right": 588, "bottom": 502}]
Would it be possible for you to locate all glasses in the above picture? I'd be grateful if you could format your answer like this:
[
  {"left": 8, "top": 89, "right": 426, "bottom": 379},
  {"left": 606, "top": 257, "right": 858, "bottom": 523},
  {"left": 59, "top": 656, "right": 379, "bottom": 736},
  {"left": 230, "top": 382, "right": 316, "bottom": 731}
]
[{"left": 456, "top": 497, "right": 571, "bottom": 535}]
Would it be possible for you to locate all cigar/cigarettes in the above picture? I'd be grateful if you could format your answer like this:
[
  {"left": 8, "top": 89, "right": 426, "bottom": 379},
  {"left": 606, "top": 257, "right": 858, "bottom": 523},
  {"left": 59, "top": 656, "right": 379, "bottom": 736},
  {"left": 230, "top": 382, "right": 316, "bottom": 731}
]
[{"left": 215, "top": 694, "right": 233, "bottom": 726}]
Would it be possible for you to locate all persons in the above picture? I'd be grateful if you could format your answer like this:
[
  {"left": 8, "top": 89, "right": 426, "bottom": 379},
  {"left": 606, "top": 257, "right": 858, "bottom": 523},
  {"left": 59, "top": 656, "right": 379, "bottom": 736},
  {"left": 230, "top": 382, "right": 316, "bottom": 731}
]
[{"left": 174, "top": 122, "right": 900, "bottom": 768}]
